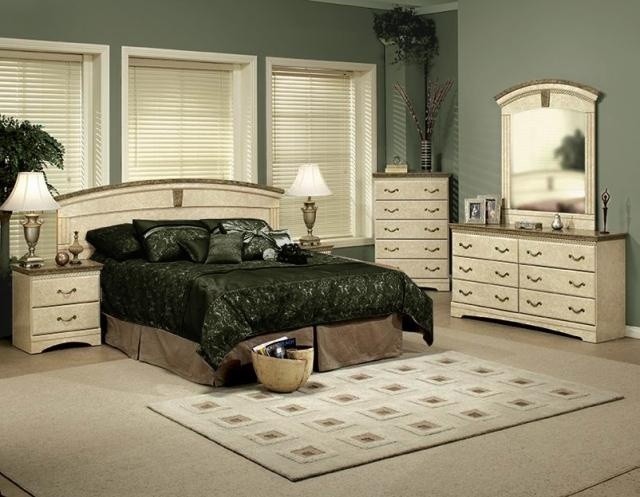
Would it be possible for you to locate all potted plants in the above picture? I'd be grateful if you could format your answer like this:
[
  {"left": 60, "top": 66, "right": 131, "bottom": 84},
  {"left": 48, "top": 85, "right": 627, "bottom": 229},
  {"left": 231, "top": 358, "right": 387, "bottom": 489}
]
[{"left": 369, "top": 3, "right": 454, "bottom": 172}]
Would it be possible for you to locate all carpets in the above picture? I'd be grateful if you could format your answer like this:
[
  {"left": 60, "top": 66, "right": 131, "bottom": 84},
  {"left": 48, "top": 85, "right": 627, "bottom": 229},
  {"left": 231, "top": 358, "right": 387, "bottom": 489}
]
[{"left": 145, "top": 351, "right": 625, "bottom": 482}]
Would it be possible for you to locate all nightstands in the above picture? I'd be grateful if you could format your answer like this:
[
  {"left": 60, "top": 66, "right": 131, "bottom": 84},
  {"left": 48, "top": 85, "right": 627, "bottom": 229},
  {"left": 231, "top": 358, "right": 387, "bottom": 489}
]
[
  {"left": 295, "top": 243, "right": 335, "bottom": 256},
  {"left": 8, "top": 259, "right": 105, "bottom": 354}
]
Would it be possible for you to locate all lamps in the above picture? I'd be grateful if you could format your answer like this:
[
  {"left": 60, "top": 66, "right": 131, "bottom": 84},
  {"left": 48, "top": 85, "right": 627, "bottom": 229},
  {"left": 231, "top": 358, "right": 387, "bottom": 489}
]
[
  {"left": 285, "top": 162, "right": 333, "bottom": 245},
  {"left": 1, "top": 172, "right": 61, "bottom": 267}
]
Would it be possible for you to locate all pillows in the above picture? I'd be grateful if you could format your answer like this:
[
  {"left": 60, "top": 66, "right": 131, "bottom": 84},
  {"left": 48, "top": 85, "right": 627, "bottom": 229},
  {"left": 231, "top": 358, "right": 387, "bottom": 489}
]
[{"left": 85, "top": 217, "right": 292, "bottom": 266}]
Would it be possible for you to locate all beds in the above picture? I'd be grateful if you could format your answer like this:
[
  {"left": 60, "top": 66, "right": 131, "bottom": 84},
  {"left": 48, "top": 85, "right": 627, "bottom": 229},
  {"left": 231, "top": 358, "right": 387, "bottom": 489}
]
[{"left": 53, "top": 178, "right": 433, "bottom": 387}]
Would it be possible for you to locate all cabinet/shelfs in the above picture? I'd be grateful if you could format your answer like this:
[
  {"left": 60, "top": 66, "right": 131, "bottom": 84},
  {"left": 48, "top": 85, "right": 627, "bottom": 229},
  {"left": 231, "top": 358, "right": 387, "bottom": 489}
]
[
  {"left": 447, "top": 227, "right": 518, "bottom": 343},
  {"left": 518, "top": 229, "right": 630, "bottom": 342},
  {"left": 372, "top": 173, "right": 452, "bottom": 291}
]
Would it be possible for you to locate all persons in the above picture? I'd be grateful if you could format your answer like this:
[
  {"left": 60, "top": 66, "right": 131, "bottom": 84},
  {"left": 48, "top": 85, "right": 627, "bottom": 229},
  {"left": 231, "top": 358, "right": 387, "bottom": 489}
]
[{"left": 468, "top": 203, "right": 480, "bottom": 218}]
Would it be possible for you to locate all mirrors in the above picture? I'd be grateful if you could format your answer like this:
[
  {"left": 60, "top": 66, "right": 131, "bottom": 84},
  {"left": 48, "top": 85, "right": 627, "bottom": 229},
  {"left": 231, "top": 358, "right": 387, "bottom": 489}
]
[{"left": 492, "top": 79, "right": 606, "bottom": 231}]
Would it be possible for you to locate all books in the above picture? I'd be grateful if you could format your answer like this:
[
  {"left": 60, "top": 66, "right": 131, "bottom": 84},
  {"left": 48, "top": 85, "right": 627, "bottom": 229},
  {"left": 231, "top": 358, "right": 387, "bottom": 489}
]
[
  {"left": 254, "top": 332, "right": 298, "bottom": 360},
  {"left": 383, "top": 159, "right": 409, "bottom": 173}
]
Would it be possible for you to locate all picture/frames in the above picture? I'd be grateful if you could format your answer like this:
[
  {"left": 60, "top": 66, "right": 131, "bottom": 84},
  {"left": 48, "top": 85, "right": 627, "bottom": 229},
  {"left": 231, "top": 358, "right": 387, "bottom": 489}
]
[
  {"left": 465, "top": 198, "right": 486, "bottom": 224},
  {"left": 477, "top": 194, "right": 501, "bottom": 224}
]
